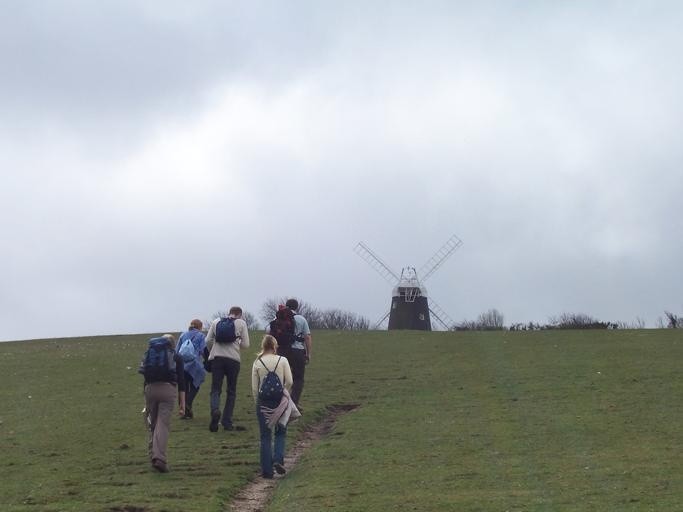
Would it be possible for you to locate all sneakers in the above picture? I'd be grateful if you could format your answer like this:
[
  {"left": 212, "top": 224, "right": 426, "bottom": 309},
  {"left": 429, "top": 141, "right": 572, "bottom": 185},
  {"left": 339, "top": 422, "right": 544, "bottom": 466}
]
[
  {"left": 208, "top": 410, "right": 220, "bottom": 433},
  {"left": 222, "top": 425, "right": 232, "bottom": 430},
  {"left": 273, "top": 460, "right": 286, "bottom": 475},
  {"left": 151, "top": 459, "right": 169, "bottom": 473}
]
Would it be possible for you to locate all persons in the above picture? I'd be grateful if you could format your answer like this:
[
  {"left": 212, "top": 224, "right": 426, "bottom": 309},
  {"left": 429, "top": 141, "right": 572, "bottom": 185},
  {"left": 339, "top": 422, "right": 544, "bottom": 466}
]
[
  {"left": 137, "top": 333, "right": 188, "bottom": 474},
  {"left": 174, "top": 317, "right": 210, "bottom": 418},
  {"left": 250, "top": 334, "right": 293, "bottom": 480},
  {"left": 204, "top": 305, "right": 250, "bottom": 431},
  {"left": 269, "top": 299, "right": 312, "bottom": 411}
]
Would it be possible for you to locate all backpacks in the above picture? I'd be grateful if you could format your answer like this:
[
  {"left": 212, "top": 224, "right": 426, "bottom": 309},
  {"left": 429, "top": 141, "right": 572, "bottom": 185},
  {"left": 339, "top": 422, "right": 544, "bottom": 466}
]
[
  {"left": 178, "top": 332, "right": 198, "bottom": 362},
  {"left": 257, "top": 356, "right": 283, "bottom": 402},
  {"left": 137, "top": 337, "right": 173, "bottom": 376},
  {"left": 213, "top": 317, "right": 241, "bottom": 344},
  {"left": 269, "top": 304, "right": 303, "bottom": 355}
]
